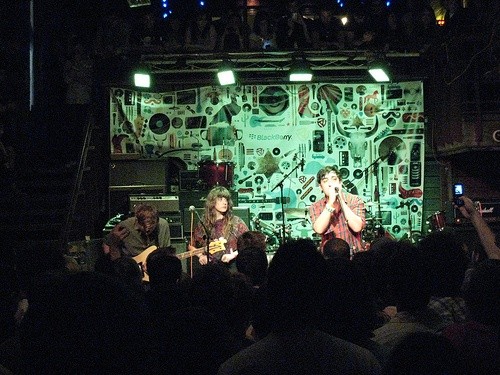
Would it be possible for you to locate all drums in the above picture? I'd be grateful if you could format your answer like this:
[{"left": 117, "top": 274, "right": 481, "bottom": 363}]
[
  {"left": 197, "top": 158, "right": 234, "bottom": 187},
  {"left": 426, "top": 210, "right": 445, "bottom": 232}
]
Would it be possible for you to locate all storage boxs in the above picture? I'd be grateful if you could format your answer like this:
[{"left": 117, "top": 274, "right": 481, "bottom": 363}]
[{"left": 180, "top": 170, "right": 199, "bottom": 192}]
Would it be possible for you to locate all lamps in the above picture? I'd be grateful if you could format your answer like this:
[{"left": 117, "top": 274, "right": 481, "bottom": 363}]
[
  {"left": 288, "top": 51, "right": 314, "bottom": 83},
  {"left": 132, "top": 56, "right": 154, "bottom": 91},
  {"left": 215, "top": 53, "right": 236, "bottom": 87},
  {"left": 366, "top": 47, "right": 394, "bottom": 83}
]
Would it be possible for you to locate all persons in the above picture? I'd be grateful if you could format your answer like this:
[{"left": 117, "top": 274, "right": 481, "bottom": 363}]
[
  {"left": 190, "top": 187, "right": 250, "bottom": 265},
  {"left": 102, "top": 204, "right": 170, "bottom": 281},
  {"left": 63, "top": 0, "right": 440, "bottom": 103},
  {"left": 0, "top": 196, "right": 500, "bottom": 374},
  {"left": 308, "top": 167, "right": 364, "bottom": 260}
]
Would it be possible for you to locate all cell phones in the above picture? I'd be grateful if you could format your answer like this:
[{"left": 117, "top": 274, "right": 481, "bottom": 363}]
[{"left": 452, "top": 183, "right": 464, "bottom": 206}]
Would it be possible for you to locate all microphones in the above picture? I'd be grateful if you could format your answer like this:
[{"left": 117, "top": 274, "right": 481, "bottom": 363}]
[
  {"left": 389, "top": 150, "right": 394, "bottom": 158},
  {"left": 335, "top": 187, "right": 339, "bottom": 192},
  {"left": 189, "top": 206, "right": 203, "bottom": 224},
  {"left": 301, "top": 154, "right": 304, "bottom": 172}
]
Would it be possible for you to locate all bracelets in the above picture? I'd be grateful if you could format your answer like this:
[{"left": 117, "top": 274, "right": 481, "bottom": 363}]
[{"left": 469, "top": 210, "right": 479, "bottom": 220}]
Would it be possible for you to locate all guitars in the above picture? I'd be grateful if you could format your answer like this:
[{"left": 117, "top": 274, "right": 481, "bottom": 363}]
[{"left": 130, "top": 236, "right": 228, "bottom": 282}]
